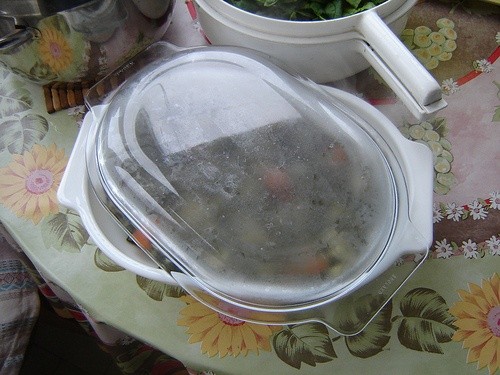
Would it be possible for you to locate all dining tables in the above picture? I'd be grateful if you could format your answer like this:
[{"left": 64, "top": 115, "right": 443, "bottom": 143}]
[{"left": 0, "top": 0, "right": 500, "bottom": 375}]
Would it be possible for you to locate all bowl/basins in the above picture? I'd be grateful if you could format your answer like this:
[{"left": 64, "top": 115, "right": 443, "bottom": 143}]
[
  {"left": 55, "top": 105, "right": 433, "bottom": 306},
  {"left": 194, "top": 0, "right": 448, "bottom": 120},
  {"left": 0, "top": 0, "right": 175, "bottom": 88}
]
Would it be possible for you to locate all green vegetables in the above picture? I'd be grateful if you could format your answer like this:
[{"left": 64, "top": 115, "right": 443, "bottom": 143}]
[{"left": 224, "top": 0, "right": 388, "bottom": 22}]
